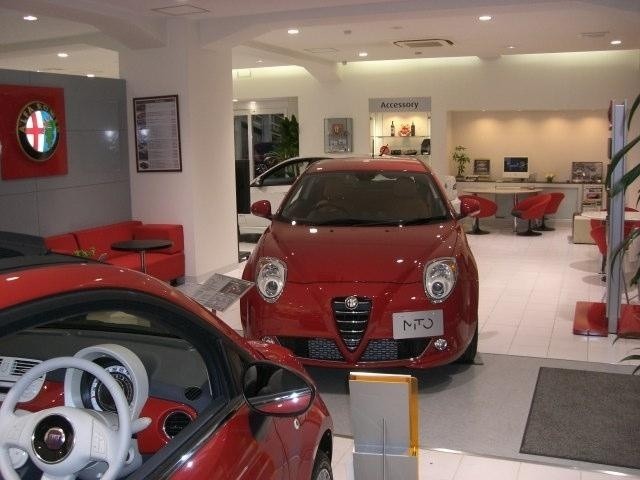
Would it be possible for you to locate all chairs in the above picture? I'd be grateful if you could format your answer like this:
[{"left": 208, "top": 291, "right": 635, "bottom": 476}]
[
  {"left": 511, "top": 194, "right": 551, "bottom": 236},
  {"left": 458, "top": 195, "right": 497, "bottom": 235},
  {"left": 534, "top": 192, "right": 565, "bottom": 231}
]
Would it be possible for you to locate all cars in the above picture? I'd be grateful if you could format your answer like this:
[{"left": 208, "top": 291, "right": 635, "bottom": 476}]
[
  {"left": 0, "top": 232, "right": 333, "bottom": 480},
  {"left": 237, "top": 154, "right": 461, "bottom": 242},
  {"left": 240, "top": 156, "right": 481, "bottom": 377},
  {"left": 242, "top": 142, "right": 299, "bottom": 181}
]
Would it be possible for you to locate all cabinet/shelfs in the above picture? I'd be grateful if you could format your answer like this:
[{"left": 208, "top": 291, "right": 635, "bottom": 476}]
[
  {"left": 456, "top": 182, "right": 608, "bottom": 221},
  {"left": 369, "top": 96, "right": 431, "bottom": 167}
]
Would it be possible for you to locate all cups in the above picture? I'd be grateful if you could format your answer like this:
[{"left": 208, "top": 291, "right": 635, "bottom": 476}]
[{"left": 391, "top": 150, "right": 401, "bottom": 155}]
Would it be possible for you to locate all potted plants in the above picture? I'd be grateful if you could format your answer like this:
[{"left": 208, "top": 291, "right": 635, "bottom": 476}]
[{"left": 448, "top": 145, "right": 474, "bottom": 181}]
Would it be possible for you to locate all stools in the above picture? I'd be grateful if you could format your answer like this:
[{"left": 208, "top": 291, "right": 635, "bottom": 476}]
[{"left": 574, "top": 216, "right": 601, "bottom": 244}]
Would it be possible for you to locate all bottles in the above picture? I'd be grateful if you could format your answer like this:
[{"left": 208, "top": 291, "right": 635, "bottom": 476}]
[
  {"left": 411, "top": 121, "right": 415, "bottom": 136},
  {"left": 391, "top": 121, "right": 395, "bottom": 136}
]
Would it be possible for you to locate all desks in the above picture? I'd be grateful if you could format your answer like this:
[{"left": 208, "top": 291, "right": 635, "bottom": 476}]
[
  {"left": 462, "top": 187, "right": 544, "bottom": 232},
  {"left": 111, "top": 239, "right": 173, "bottom": 273},
  {"left": 581, "top": 212, "right": 640, "bottom": 274}
]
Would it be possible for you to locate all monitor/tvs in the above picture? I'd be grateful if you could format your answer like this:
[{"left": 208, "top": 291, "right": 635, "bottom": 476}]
[
  {"left": 502, "top": 156, "right": 529, "bottom": 182},
  {"left": 474, "top": 159, "right": 491, "bottom": 175}
]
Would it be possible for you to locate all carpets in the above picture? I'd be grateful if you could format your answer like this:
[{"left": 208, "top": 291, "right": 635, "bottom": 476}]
[{"left": 519, "top": 367, "right": 640, "bottom": 469}]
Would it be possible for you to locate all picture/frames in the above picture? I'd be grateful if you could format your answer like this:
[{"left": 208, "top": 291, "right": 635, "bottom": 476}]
[
  {"left": 133, "top": 95, "right": 182, "bottom": 172},
  {"left": 572, "top": 162, "right": 603, "bottom": 183}
]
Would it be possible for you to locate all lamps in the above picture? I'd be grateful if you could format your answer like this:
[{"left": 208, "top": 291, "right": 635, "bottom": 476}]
[{"left": 324, "top": 118, "right": 352, "bottom": 152}]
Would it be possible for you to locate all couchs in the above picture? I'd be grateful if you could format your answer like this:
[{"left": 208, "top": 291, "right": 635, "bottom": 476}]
[{"left": 45, "top": 221, "right": 185, "bottom": 287}]
